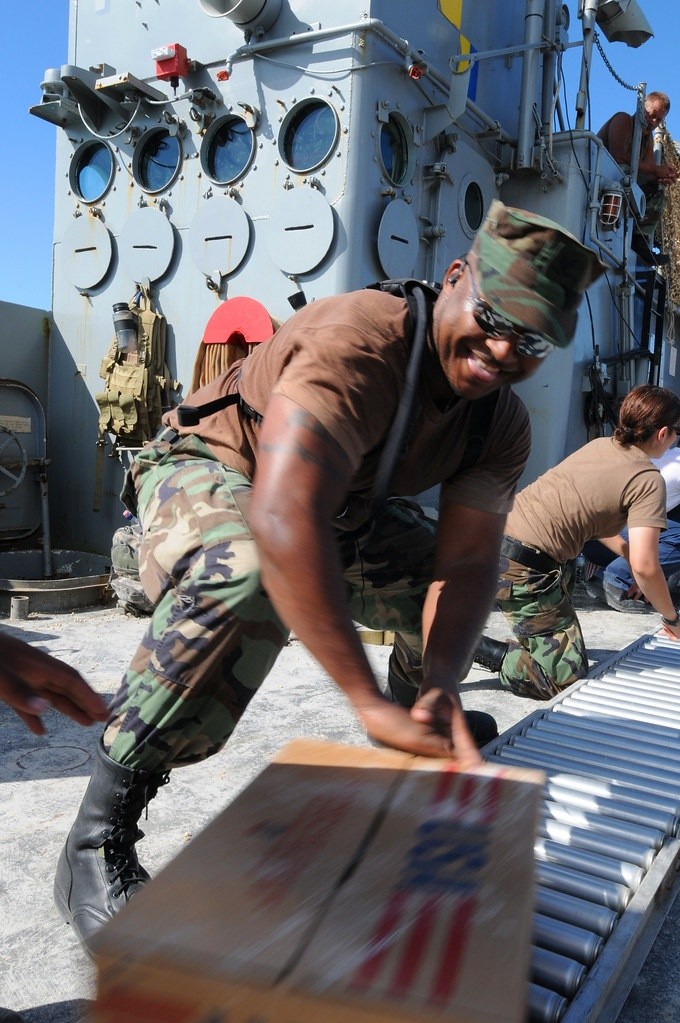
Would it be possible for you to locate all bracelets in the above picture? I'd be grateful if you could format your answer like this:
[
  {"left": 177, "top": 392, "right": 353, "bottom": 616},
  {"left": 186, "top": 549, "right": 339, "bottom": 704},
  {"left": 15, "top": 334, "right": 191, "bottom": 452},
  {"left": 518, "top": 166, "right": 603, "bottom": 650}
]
[{"left": 662, "top": 613, "right": 679, "bottom": 625}]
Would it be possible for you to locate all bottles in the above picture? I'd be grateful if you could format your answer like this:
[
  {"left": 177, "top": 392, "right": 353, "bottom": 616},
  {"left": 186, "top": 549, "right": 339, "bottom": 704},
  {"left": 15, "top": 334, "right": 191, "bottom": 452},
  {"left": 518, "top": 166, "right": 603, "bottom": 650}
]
[{"left": 575, "top": 552, "right": 586, "bottom": 583}]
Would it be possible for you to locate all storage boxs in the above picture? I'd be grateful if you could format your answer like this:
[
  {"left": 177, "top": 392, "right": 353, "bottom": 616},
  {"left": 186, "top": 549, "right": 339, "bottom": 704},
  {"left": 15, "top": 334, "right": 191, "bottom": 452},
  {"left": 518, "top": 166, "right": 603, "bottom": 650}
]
[{"left": 83, "top": 737, "right": 547, "bottom": 1023}]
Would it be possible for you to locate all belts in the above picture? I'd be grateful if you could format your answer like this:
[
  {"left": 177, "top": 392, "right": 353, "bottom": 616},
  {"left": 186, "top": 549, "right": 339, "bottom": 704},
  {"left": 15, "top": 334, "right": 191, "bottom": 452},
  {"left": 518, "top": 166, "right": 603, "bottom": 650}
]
[
  {"left": 156, "top": 424, "right": 182, "bottom": 448},
  {"left": 499, "top": 535, "right": 562, "bottom": 576}
]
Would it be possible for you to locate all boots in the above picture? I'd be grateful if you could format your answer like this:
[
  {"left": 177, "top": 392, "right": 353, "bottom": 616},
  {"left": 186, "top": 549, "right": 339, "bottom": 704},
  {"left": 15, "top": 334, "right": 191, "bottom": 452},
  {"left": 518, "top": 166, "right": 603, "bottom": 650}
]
[
  {"left": 54, "top": 734, "right": 172, "bottom": 966},
  {"left": 472, "top": 635, "right": 509, "bottom": 673},
  {"left": 368, "top": 654, "right": 499, "bottom": 749}
]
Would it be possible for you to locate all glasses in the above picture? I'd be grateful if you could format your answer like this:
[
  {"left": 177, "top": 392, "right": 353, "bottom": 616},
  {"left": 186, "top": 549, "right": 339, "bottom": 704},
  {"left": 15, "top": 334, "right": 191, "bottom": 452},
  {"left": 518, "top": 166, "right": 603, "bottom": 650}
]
[
  {"left": 465, "top": 259, "right": 553, "bottom": 359},
  {"left": 666, "top": 423, "right": 680, "bottom": 435}
]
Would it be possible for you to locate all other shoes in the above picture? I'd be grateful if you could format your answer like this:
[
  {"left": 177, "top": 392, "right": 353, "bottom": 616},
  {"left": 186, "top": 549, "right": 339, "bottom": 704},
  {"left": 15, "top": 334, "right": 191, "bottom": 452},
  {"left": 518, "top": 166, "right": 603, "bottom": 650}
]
[
  {"left": 651, "top": 252, "right": 669, "bottom": 265},
  {"left": 631, "top": 232, "right": 656, "bottom": 266}
]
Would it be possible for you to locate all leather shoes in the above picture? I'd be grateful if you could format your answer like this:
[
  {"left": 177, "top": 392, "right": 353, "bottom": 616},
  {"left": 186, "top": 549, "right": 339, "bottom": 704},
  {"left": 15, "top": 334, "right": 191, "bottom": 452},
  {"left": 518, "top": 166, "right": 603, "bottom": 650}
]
[{"left": 586, "top": 575, "right": 647, "bottom": 614}]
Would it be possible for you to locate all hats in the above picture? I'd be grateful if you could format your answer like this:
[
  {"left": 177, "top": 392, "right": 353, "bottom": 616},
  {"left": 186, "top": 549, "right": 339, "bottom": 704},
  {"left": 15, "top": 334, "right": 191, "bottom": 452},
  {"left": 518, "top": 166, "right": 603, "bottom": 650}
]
[{"left": 467, "top": 198, "right": 612, "bottom": 349}]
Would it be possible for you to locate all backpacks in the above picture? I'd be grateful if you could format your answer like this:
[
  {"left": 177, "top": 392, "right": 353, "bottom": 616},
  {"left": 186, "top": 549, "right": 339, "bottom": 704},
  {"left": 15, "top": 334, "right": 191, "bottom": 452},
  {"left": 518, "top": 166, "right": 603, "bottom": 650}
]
[{"left": 104, "top": 523, "right": 156, "bottom": 618}]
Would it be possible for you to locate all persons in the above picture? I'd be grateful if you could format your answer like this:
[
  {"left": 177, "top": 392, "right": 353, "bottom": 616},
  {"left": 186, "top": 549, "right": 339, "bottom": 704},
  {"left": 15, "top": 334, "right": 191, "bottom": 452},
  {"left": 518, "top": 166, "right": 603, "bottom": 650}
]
[
  {"left": 0, "top": 631, "right": 110, "bottom": 735},
  {"left": 54, "top": 200, "right": 611, "bottom": 966},
  {"left": 597, "top": 91, "right": 680, "bottom": 264},
  {"left": 581, "top": 439, "right": 680, "bottom": 614},
  {"left": 474, "top": 384, "right": 680, "bottom": 700}
]
